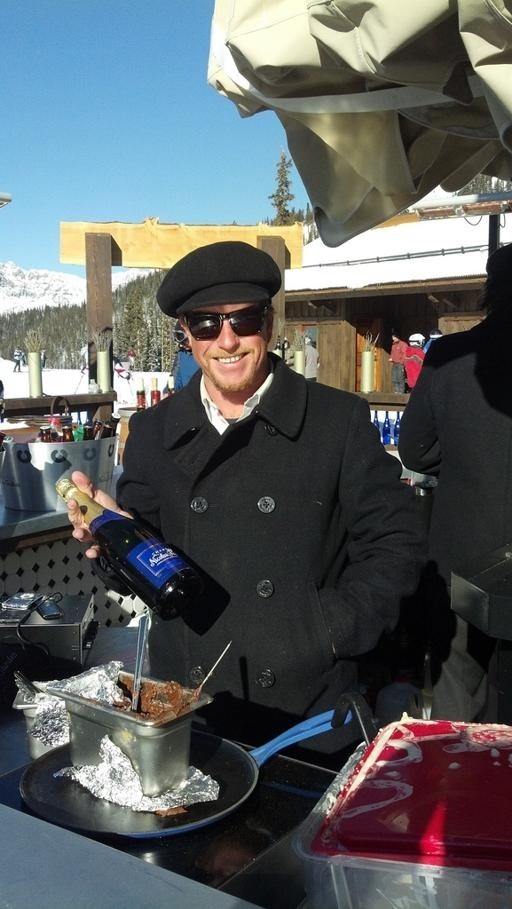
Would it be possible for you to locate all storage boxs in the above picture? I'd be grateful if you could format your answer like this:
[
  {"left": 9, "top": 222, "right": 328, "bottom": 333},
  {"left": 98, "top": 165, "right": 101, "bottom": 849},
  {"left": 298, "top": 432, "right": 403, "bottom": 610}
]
[{"left": 292, "top": 719, "right": 511, "bottom": 909}]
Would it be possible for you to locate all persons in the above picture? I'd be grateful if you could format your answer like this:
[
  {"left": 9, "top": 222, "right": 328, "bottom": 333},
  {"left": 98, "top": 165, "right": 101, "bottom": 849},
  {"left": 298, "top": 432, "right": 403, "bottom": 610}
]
[
  {"left": 79, "top": 344, "right": 89, "bottom": 375},
  {"left": 64, "top": 241, "right": 429, "bottom": 760},
  {"left": 302, "top": 337, "right": 321, "bottom": 383},
  {"left": 388, "top": 333, "right": 408, "bottom": 393},
  {"left": 284, "top": 340, "right": 295, "bottom": 370},
  {"left": 12, "top": 346, "right": 22, "bottom": 373},
  {"left": 403, "top": 333, "right": 427, "bottom": 391},
  {"left": 423, "top": 329, "right": 443, "bottom": 353},
  {"left": 395, "top": 240, "right": 512, "bottom": 727}
]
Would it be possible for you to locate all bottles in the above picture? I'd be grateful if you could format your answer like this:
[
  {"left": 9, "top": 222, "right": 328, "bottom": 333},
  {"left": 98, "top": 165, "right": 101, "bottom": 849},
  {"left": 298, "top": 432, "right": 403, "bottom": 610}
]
[
  {"left": 150, "top": 376, "right": 161, "bottom": 407},
  {"left": 167, "top": 374, "right": 176, "bottom": 395},
  {"left": 87, "top": 379, "right": 99, "bottom": 394},
  {"left": 372, "top": 410, "right": 381, "bottom": 446},
  {"left": 393, "top": 410, "right": 402, "bottom": 447},
  {"left": 382, "top": 410, "right": 391, "bottom": 445},
  {"left": 274, "top": 332, "right": 283, "bottom": 360},
  {"left": 136, "top": 377, "right": 146, "bottom": 411},
  {"left": 53, "top": 477, "right": 201, "bottom": 622},
  {"left": 38, "top": 412, "right": 122, "bottom": 444}
]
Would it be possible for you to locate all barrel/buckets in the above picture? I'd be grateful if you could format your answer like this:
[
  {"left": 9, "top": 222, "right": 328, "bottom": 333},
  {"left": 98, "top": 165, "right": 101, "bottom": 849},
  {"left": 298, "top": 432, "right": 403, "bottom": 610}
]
[{"left": 0, "top": 424, "right": 119, "bottom": 511}]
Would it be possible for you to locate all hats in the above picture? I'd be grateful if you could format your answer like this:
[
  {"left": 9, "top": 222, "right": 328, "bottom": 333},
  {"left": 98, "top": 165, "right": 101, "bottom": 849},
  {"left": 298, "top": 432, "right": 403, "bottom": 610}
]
[
  {"left": 486, "top": 244, "right": 512, "bottom": 273},
  {"left": 156, "top": 241, "right": 282, "bottom": 318}
]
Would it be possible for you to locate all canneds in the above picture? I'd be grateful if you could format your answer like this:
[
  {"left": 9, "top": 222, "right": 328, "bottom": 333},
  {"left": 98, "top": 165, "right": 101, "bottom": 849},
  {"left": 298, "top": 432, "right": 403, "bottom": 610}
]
[
  {"left": 150, "top": 391, "right": 160, "bottom": 407},
  {"left": 136, "top": 390, "right": 145, "bottom": 411}
]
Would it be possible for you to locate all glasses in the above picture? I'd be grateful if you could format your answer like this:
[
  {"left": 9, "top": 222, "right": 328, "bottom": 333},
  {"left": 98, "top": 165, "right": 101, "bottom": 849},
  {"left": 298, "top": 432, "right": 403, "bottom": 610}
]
[{"left": 183, "top": 308, "right": 272, "bottom": 339}]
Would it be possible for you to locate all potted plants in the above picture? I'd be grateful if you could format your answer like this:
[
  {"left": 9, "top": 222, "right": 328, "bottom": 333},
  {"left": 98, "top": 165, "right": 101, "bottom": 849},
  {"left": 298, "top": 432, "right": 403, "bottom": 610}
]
[
  {"left": 360, "top": 331, "right": 379, "bottom": 394},
  {"left": 292, "top": 331, "right": 305, "bottom": 375},
  {"left": 23, "top": 331, "right": 41, "bottom": 397},
  {"left": 91, "top": 329, "right": 112, "bottom": 393}
]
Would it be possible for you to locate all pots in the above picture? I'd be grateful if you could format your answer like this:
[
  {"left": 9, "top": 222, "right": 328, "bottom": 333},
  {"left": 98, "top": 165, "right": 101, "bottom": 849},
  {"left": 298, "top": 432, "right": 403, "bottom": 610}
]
[{"left": 16, "top": 699, "right": 357, "bottom": 845}]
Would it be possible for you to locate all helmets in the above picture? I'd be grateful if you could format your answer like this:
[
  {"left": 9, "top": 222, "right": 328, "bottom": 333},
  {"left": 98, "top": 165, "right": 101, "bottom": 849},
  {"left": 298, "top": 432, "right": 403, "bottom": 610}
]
[{"left": 409, "top": 334, "right": 426, "bottom": 348}]
